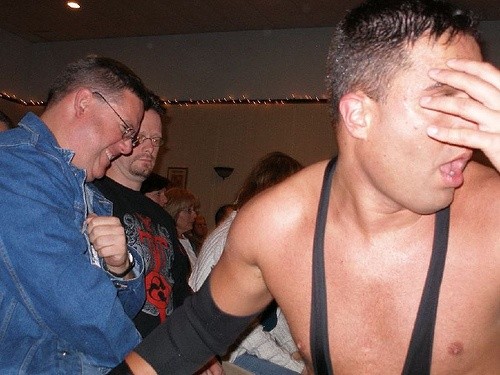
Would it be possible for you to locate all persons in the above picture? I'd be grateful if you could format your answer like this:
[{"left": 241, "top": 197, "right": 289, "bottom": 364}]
[
  {"left": 0, "top": 52, "right": 153, "bottom": 375},
  {"left": 0, "top": 110, "right": 13, "bottom": 131},
  {"left": 92, "top": 0, "right": 500, "bottom": 375}
]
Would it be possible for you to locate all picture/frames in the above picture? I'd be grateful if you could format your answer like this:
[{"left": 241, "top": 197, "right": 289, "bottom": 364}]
[{"left": 167, "top": 167, "right": 188, "bottom": 188}]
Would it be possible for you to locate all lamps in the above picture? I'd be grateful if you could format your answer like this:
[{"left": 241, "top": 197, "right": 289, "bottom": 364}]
[{"left": 214, "top": 167, "right": 233, "bottom": 180}]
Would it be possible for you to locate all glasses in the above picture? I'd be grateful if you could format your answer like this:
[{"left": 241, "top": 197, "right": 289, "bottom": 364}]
[
  {"left": 133, "top": 133, "right": 164, "bottom": 146},
  {"left": 92, "top": 91, "right": 137, "bottom": 141}
]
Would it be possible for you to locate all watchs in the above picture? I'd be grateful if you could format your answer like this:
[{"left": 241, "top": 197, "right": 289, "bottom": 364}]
[{"left": 103, "top": 249, "right": 136, "bottom": 278}]
